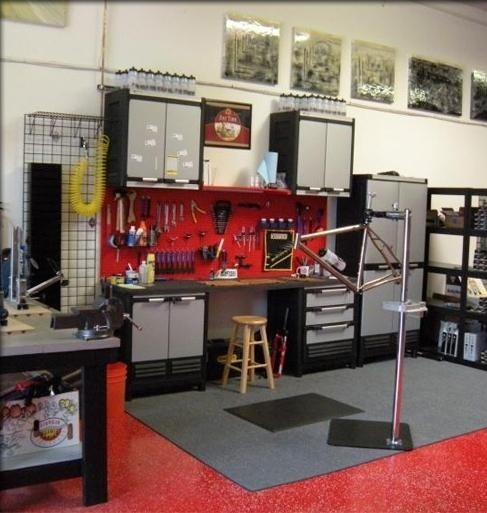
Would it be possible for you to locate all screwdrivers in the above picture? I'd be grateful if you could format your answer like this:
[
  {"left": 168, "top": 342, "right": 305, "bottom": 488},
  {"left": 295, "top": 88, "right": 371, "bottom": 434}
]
[{"left": 233, "top": 225, "right": 256, "bottom": 251}]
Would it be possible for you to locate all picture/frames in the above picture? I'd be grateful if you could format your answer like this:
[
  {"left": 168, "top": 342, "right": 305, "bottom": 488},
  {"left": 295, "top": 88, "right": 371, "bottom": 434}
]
[{"left": 203, "top": 97, "right": 252, "bottom": 147}]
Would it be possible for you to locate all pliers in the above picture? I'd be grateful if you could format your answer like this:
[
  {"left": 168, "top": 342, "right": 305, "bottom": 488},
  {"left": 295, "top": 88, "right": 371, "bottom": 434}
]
[{"left": 190, "top": 198, "right": 207, "bottom": 224}]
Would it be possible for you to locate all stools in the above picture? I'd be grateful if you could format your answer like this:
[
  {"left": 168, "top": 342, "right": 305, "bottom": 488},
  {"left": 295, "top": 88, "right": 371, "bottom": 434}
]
[{"left": 220, "top": 316, "right": 278, "bottom": 395}]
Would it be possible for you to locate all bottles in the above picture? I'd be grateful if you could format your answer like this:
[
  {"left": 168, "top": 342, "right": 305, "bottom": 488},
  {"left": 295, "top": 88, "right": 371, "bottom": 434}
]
[
  {"left": 138, "top": 260, "right": 147, "bottom": 284},
  {"left": 278, "top": 90, "right": 349, "bottom": 116},
  {"left": 114, "top": 65, "right": 197, "bottom": 97},
  {"left": 127, "top": 226, "right": 136, "bottom": 247},
  {"left": 138, "top": 221, "right": 147, "bottom": 246}
]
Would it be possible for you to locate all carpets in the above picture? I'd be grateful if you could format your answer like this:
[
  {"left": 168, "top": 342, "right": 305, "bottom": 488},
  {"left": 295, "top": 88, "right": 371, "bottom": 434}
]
[
  {"left": 326, "top": 412, "right": 414, "bottom": 452},
  {"left": 223, "top": 391, "right": 365, "bottom": 435},
  {"left": 124, "top": 353, "right": 487, "bottom": 492}
]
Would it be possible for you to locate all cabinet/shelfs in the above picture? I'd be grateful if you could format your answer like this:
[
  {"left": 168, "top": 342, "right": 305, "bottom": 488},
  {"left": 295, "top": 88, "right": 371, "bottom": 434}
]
[
  {"left": 271, "top": 111, "right": 355, "bottom": 200},
  {"left": 107, "top": 281, "right": 207, "bottom": 398},
  {"left": 417, "top": 186, "right": 487, "bottom": 370},
  {"left": 335, "top": 170, "right": 429, "bottom": 265},
  {"left": 335, "top": 266, "right": 428, "bottom": 366},
  {"left": 104, "top": 89, "right": 202, "bottom": 191}
]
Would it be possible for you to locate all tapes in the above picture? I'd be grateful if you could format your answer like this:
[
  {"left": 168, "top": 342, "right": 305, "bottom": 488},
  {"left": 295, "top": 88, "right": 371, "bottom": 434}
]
[{"left": 110, "top": 234, "right": 117, "bottom": 247}]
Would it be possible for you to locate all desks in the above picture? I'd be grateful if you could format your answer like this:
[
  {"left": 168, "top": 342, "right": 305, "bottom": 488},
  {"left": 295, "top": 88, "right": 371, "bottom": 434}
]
[
  {"left": 0, "top": 289, "right": 122, "bottom": 507},
  {"left": 201, "top": 274, "right": 359, "bottom": 375}
]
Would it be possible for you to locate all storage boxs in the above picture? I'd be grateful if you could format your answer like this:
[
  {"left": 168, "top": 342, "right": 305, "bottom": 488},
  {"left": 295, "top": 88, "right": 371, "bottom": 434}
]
[
  {"left": 441, "top": 206, "right": 478, "bottom": 228},
  {"left": 1, "top": 373, "right": 80, "bottom": 457}
]
